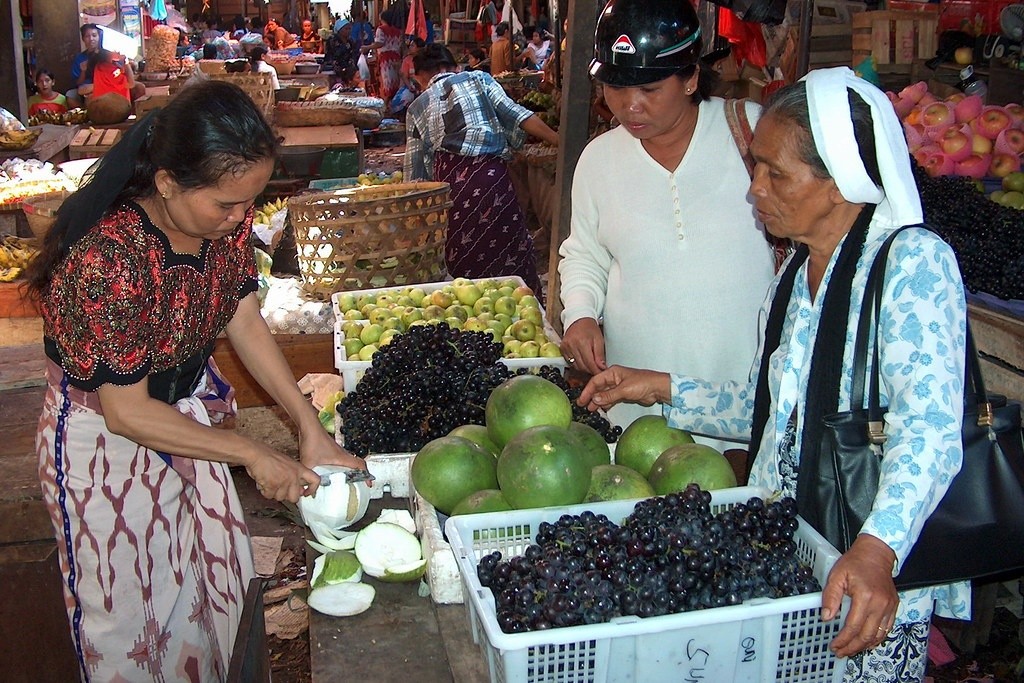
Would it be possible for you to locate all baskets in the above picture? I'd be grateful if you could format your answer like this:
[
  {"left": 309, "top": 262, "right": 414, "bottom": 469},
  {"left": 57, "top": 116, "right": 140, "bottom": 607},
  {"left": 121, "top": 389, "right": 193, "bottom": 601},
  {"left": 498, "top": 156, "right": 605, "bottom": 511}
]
[
  {"left": 23, "top": 192, "right": 73, "bottom": 239},
  {"left": 443, "top": 484, "right": 850, "bottom": 682}
]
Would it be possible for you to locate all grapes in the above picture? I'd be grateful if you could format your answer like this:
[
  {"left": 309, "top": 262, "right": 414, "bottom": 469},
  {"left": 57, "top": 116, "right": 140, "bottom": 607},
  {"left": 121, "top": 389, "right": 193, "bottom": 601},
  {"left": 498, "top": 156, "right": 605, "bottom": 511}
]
[
  {"left": 334, "top": 321, "right": 623, "bottom": 461},
  {"left": 477, "top": 481, "right": 842, "bottom": 683},
  {"left": 908, "top": 153, "right": 1024, "bottom": 302}
]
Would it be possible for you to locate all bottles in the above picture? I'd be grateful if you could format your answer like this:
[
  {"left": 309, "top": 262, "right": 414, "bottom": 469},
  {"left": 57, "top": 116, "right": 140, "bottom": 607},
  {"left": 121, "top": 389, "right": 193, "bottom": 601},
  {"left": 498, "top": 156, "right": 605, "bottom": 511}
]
[{"left": 960, "top": 65, "right": 987, "bottom": 106}]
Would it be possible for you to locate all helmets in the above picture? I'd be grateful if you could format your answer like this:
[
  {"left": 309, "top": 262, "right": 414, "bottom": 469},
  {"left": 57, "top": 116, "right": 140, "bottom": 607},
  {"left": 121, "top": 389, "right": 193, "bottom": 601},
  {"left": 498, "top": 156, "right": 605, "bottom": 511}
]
[{"left": 587, "top": 0, "right": 703, "bottom": 85}]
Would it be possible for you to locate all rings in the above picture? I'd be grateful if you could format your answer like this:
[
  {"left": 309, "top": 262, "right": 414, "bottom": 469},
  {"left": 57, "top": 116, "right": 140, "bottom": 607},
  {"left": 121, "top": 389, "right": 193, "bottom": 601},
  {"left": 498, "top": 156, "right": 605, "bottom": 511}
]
[
  {"left": 878, "top": 627, "right": 885, "bottom": 632},
  {"left": 568, "top": 359, "right": 575, "bottom": 363},
  {"left": 885, "top": 629, "right": 890, "bottom": 635}
]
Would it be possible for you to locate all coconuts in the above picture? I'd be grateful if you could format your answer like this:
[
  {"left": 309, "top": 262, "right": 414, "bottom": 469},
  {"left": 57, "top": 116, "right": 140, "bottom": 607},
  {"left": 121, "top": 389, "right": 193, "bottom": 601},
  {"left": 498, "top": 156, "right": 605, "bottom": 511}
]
[
  {"left": 409, "top": 374, "right": 735, "bottom": 540},
  {"left": 297, "top": 465, "right": 370, "bottom": 532}
]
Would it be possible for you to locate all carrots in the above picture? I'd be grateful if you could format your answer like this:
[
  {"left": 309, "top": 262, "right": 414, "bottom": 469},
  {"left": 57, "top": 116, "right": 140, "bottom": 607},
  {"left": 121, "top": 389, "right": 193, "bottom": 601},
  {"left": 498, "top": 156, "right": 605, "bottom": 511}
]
[{"left": 143, "top": 27, "right": 195, "bottom": 73}]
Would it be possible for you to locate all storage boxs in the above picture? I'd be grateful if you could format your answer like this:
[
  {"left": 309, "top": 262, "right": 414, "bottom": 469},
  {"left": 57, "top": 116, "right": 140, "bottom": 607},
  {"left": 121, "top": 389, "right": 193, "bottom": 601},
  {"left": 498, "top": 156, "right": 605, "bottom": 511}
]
[
  {"left": 134, "top": 93, "right": 178, "bottom": 121},
  {"left": 851, "top": 11, "right": 940, "bottom": 73},
  {"left": 442, "top": 18, "right": 477, "bottom": 44},
  {"left": 332, "top": 276, "right": 851, "bottom": 683},
  {"left": 69, "top": 128, "right": 122, "bottom": 160}
]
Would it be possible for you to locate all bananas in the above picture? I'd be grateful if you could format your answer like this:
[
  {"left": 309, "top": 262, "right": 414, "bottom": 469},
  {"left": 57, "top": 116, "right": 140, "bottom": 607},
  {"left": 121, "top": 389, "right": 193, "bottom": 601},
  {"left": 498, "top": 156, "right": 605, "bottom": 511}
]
[
  {"left": 0, "top": 239, "right": 40, "bottom": 282},
  {"left": 252, "top": 196, "right": 289, "bottom": 224},
  {"left": 31, "top": 108, "right": 87, "bottom": 126},
  {"left": 0, "top": 127, "right": 44, "bottom": 149}
]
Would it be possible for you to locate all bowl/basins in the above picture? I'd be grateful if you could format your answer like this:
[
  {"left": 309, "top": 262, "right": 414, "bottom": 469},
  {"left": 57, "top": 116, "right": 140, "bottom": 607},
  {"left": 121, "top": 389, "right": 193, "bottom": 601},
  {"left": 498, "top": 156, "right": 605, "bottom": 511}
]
[
  {"left": 287, "top": 48, "right": 303, "bottom": 56},
  {"left": 276, "top": 146, "right": 326, "bottom": 176},
  {"left": 295, "top": 63, "right": 320, "bottom": 74},
  {"left": 0, "top": 130, "right": 38, "bottom": 151}
]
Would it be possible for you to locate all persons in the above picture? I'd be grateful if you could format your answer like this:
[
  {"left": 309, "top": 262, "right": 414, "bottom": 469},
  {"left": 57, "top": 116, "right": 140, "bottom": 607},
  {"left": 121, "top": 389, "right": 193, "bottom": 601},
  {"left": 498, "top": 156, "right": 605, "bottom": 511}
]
[
  {"left": 172, "top": 12, "right": 320, "bottom": 89},
  {"left": 574, "top": 66, "right": 975, "bottom": 682},
  {"left": 319, "top": 0, "right": 434, "bottom": 123},
  {"left": 402, "top": 43, "right": 561, "bottom": 305},
  {"left": 65, "top": 20, "right": 146, "bottom": 107},
  {"left": 557, "top": 0, "right": 802, "bottom": 460},
  {"left": 19, "top": 77, "right": 374, "bottom": 683},
  {"left": 27, "top": 70, "right": 68, "bottom": 119},
  {"left": 463, "top": 0, "right": 568, "bottom": 89}
]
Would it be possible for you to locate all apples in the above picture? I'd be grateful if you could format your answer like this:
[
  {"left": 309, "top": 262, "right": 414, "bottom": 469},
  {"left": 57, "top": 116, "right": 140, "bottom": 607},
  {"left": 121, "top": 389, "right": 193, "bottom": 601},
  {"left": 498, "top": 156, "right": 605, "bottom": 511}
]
[
  {"left": 339, "top": 276, "right": 562, "bottom": 361},
  {"left": 955, "top": 47, "right": 972, "bottom": 65},
  {"left": 355, "top": 169, "right": 404, "bottom": 186},
  {"left": 517, "top": 90, "right": 554, "bottom": 108},
  {"left": 884, "top": 81, "right": 1024, "bottom": 210}
]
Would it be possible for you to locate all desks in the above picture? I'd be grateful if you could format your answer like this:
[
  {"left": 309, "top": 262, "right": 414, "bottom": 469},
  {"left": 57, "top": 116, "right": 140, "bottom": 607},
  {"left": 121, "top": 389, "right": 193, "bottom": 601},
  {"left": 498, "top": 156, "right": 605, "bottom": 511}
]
[
  {"left": 0, "top": 124, "right": 80, "bottom": 165},
  {"left": 306, "top": 496, "right": 489, "bottom": 683},
  {"left": 276, "top": 122, "right": 364, "bottom": 176}
]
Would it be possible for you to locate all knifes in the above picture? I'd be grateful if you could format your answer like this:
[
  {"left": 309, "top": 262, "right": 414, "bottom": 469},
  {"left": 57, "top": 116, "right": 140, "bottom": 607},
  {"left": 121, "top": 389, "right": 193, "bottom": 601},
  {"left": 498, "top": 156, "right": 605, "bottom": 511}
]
[{"left": 255, "top": 471, "right": 376, "bottom": 491}]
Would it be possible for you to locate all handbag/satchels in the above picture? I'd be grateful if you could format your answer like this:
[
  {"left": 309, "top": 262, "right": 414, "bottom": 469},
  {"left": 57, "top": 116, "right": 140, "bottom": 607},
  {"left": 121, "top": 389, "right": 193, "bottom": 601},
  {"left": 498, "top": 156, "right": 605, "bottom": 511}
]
[
  {"left": 818, "top": 224, "right": 1024, "bottom": 591},
  {"left": 358, "top": 51, "right": 371, "bottom": 81}
]
